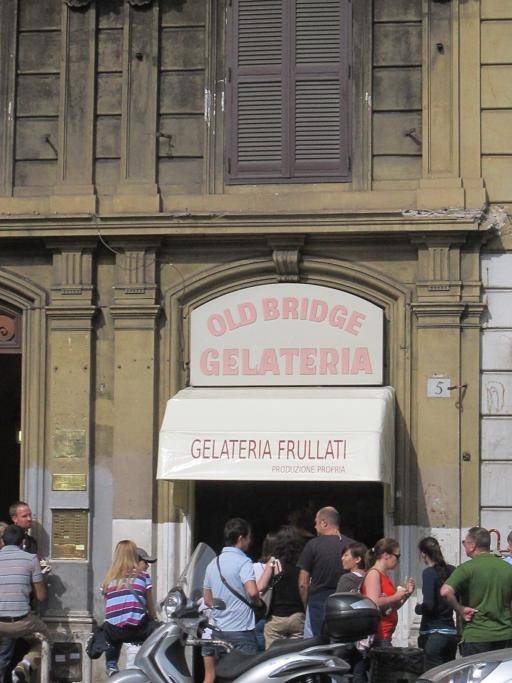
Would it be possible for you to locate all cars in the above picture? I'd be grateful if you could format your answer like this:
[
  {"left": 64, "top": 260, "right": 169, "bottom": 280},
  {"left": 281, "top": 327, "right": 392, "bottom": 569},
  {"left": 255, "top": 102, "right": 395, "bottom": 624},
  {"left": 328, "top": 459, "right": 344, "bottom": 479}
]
[{"left": 417, "top": 647, "right": 512, "bottom": 682}]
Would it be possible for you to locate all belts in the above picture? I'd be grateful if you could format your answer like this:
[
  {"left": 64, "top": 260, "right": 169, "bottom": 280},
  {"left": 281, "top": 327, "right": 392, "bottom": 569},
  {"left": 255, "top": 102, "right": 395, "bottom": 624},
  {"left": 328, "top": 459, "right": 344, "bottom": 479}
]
[{"left": 0, "top": 613, "right": 30, "bottom": 623}]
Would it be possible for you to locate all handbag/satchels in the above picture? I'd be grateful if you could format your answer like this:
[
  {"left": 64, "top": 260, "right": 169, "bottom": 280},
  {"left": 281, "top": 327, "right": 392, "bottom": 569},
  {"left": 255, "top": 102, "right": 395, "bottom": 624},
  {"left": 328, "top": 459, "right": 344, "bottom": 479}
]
[
  {"left": 252, "top": 586, "right": 275, "bottom": 623},
  {"left": 85, "top": 622, "right": 116, "bottom": 659}
]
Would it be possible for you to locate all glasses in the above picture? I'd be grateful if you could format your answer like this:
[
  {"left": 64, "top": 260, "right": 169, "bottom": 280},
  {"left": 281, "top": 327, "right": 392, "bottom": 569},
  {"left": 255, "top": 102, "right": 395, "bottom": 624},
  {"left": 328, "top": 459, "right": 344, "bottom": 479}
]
[{"left": 392, "top": 550, "right": 402, "bottom": 561}]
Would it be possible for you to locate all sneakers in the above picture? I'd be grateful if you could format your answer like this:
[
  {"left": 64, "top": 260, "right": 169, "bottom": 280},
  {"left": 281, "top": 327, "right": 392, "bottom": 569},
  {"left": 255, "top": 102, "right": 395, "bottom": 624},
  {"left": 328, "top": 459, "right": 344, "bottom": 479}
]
[
  {"left": 15, "top": 662, "right": 32, "bottom": 683},
  {"left": 105, "top": 659, "right": 119, "bottom": 677}
]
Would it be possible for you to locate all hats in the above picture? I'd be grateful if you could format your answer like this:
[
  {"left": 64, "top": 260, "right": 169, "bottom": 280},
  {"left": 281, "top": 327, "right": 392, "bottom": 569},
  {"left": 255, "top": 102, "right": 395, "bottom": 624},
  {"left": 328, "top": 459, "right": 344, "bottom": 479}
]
[{"left": 135, "top": 547, "right": 157, "bottom": 565}]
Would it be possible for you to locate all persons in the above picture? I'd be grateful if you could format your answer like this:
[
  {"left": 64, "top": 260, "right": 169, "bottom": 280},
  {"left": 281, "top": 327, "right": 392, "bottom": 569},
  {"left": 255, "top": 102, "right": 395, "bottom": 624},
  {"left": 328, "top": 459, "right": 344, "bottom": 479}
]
[
  {"left": 102, "top": 540, "right": 166, "bottom": 676},
  {"left": 125, "top": 548, "right": 158, "bottom": 669},
  {"left": 251, "top": 530, "right": 279, "bottom": 653},
  {"left": 202, "top": 518, "right": 262, "bottom": 657},
  {"left": 262, "top": 525, "right": 316, "bottom": 649},
  {"left": 361, "top": 536, "right": 414, "bottom": 648},
  {"left": 438, "top": 527, "right": 512, "bottom": 658},
  {"left": 0, "top": 522, "right": 55, "bottom": 683},
  {"left": 297, "top": 505, "right": 355, "bottom": 637},
  {"left": 502, "top": 530, "right": 512, "bottom": 565},
  {"left": 334, "top": 539, "right": 366, "bottom": 593},
  {"left": 8, "top": 502, "right": 39, "bottom": 553},
  {"left": 198, "top": 597, "right": 221, "bottom": 683},
  {"left": 1, "top": 520, "right": 9, "bottom": 548},
  {"left": 417, "top": 536, "right": 461, "bottom": 673}
]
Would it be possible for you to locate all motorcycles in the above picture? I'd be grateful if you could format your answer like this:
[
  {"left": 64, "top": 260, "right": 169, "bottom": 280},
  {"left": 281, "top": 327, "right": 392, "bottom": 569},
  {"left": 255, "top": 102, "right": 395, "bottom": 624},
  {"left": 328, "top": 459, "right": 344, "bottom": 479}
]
[{"left": 102, "top": 540, "right": 382, "bottom": 681}]
